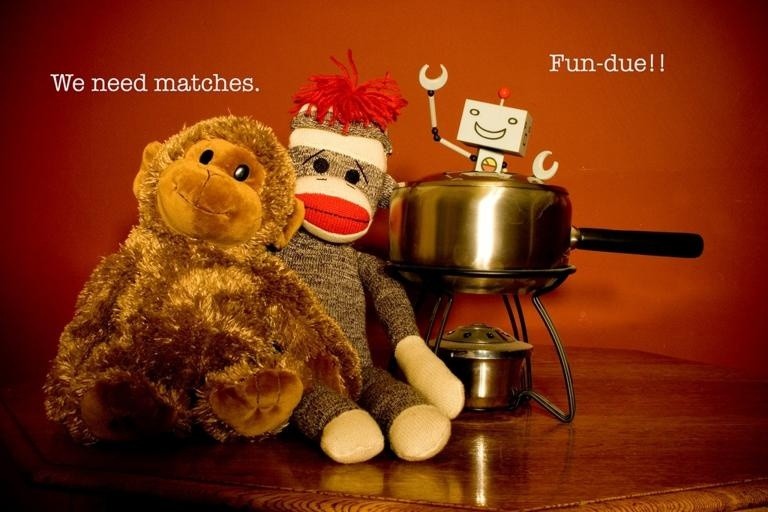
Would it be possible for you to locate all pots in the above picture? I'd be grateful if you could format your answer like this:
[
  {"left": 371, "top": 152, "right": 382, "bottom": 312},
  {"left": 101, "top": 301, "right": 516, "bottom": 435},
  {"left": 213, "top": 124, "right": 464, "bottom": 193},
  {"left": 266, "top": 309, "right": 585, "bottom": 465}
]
[{"left": 388, "top": 171, "right": 704, "bottom": 297}]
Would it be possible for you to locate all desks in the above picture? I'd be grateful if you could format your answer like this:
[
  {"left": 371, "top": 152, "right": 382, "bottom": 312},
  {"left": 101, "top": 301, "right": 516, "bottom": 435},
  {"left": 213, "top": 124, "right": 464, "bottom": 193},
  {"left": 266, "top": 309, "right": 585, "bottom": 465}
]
[{"left": 25, "top": 350, "right": 768, "bottom": 512}]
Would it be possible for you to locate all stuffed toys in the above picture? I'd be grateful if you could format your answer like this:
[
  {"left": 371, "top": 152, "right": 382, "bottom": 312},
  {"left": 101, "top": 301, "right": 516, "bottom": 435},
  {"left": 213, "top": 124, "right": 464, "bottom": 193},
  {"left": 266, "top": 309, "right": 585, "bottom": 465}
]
[
  {"left": 277, "top": 49, "right": 466, "bottom": 464},
  {"left": 41, "top": 115, "right": 359, "bottom": 447}
]
